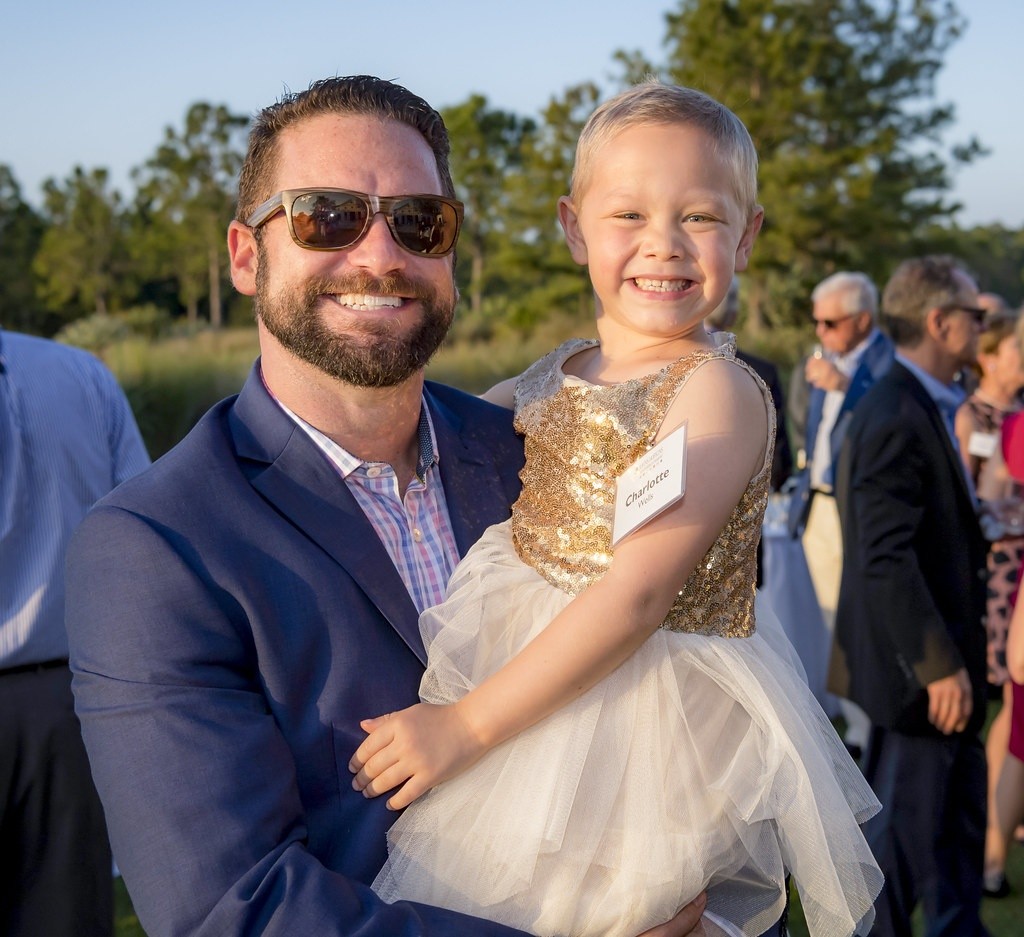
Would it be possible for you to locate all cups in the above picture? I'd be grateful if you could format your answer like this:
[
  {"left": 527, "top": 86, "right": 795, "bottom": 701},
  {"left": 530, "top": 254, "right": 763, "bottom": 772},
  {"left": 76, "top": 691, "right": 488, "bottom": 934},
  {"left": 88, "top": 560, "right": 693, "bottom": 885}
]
[
  {"left": 996, "top": 481, "right": 1024, "bottom": 534},
  {"left": 805, "top": 342, "right": 838, "bottom": 362}
]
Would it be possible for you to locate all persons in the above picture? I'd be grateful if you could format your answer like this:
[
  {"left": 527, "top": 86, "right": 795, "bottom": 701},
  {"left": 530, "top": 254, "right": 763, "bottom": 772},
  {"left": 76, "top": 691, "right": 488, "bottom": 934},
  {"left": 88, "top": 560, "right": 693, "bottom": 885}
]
[
  {"left": 1, "top": 327, "right": 153, "bottom": 936},
  {"left": 63, "top": 76, "right": 775, "bottom": 937},
  {"left": 704, "top": 253, "right": 1023, "bottom": 937},
  {"left": 348, "top": 81, "right": 887, "bottom": 937}
]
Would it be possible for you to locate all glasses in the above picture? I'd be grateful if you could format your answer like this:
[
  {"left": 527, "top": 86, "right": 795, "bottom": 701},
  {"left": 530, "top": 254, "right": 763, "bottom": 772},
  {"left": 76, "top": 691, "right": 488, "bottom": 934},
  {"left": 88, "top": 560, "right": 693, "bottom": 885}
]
[
  {"left": 810, "top": 315, "right": 853, "bottom": 329},
  {"left": 960, "top": 306, "right": 987, "bottom": 324},
  {"left": 245, "top": 186, "right": 464, "bottom": 258}
]
